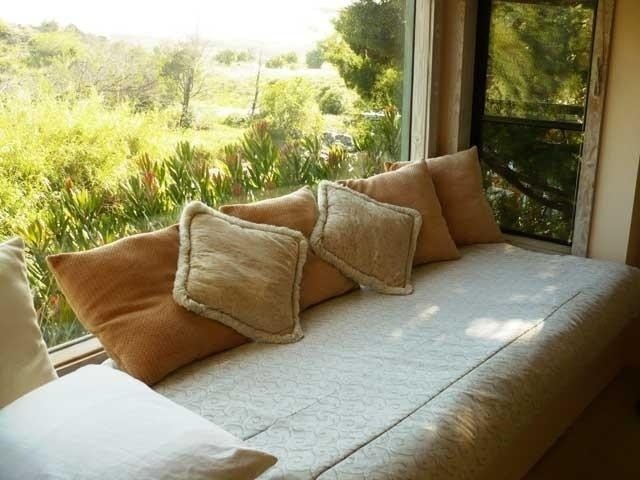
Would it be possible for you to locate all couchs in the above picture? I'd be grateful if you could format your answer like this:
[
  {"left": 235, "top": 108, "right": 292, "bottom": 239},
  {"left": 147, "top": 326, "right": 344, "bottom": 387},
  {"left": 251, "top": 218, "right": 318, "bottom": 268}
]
[{"left": 0, "top": 146, "right": 640, "bottom": 480}]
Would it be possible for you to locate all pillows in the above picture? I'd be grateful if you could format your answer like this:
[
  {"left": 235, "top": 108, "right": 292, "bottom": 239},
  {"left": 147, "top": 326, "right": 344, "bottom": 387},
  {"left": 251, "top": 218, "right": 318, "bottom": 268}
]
[
  {"left": 309, "top": 177, "right": 424, "bottom": 299},
  {"left": 340, "top": 160, "right": 461, "bottom": 267},
  {"left": 0, "top": 362, "right": 278, "bottom": 480},
  {"left": 172, "top": 201, "right": 309, "bottom": 346},
  {"left": 219, "top": 186, "right": 360, "bottom": 315},
  {"left": 383, "top": 146, "right": 506, "bottom": 243},
  {"left": 44, "top": 224, "right": 254, "bottom": 387},
  {"left": 0, "top": 236, "right": 61, "bottom": 408}
]
